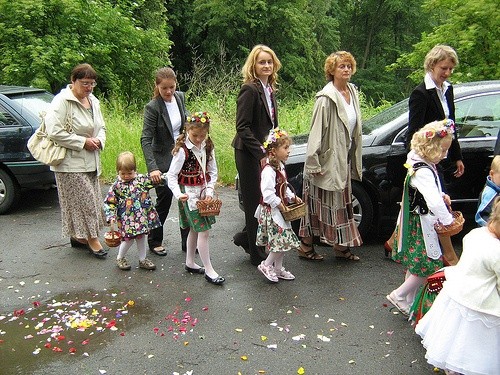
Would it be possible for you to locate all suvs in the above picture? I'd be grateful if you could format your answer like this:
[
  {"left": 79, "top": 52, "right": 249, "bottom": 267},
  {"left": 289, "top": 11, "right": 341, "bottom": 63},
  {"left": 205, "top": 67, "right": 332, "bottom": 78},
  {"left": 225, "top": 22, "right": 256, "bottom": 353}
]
[{"left": 1, "top": 83, "right": 58, "bottom": 212}]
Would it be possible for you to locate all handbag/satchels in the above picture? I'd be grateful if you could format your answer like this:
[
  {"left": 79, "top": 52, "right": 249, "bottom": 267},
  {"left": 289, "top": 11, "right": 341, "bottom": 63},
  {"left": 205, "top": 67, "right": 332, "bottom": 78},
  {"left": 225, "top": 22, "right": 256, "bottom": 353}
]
[{"left": 27, "top": 96, "right": 73, "bottom": 166}]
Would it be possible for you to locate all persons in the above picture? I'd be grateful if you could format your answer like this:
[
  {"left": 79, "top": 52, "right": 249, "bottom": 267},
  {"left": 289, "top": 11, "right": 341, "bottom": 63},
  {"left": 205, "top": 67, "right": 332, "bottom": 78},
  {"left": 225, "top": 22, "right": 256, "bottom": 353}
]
[
  {"left": 166, "top": 112, "right": 225, "bottom": 284},
  {"left": 297, "top": 51, "right": 363, "bottom": 260},
  {"left": 231, "top": 44, "right": 282, "bottom": 267},
  {"left": 45, "top": 64, "right": 107, "bottom": 257},
  {"left": 103, "top": 152, "right": 162, "bottom": 269},
  {"left": 386, "top": 119, "right": 457, "bottom": 319},
  {"left": 475, "top": 155, "right": 500, "bottom": 226},
  {"left": 385, "top": 44, "right": 464, "bottom": 257},
  {"left": 414, "top": 196, "right": 500, "bottom": 375},
  {"left": 141, "top": 68, "right": 198, "bottom": 256},
  {"left": 254, "top": 128, "right": 302, "bottom": 282}
]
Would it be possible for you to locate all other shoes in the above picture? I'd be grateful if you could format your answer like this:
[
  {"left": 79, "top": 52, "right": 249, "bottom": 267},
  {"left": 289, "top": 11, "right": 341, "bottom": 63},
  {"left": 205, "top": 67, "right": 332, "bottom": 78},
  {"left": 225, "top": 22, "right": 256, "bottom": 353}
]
[
  {"left": 116, "top": 257, "right": 131, "bottom": 270},
  {"left": 442, "top": 256, "right": 450, "bottom": 267},
  {"left": 204, "top": 271, "right": 225, "bottom": 283},
  {"left": 182, "top": 247, "right": 199, "bottom": 253},
  {"left": 251, "top": 253, "right": 269, "bottom": 266},
  {"left": 151, "top": 246, "right": 166, "bottom": 256},
  {"left": 233, "top": 239, "right": 250, "bottom": 255},
  {"left": 139, "top": 259, "right": 156, "bottom": 270},
  {"left": 185, "top": 262, "right": 205, "bottom": 273}
]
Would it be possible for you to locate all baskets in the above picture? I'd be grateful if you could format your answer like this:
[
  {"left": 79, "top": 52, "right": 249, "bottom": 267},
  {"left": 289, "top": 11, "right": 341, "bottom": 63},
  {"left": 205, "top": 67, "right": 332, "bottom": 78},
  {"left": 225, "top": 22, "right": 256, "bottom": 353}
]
[
  {"left": 432, "top": 203, "right": 464, "bottom": 236},
  {"left": 279, "top": 182, "right": 305, "bottom": 221},
  {"left": 104, "top": 220, "right": 122, "bottom": 247},
  {"left": 196, "top": 187, "right": 222, "bottom": 216}
]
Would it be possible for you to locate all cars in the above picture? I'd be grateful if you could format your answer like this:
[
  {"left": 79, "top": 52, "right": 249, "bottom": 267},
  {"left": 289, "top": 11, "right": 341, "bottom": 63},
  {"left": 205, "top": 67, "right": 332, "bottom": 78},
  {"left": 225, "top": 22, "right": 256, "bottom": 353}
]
[{"left": 234, "top": 78, "right": 500, "bottom": 244}]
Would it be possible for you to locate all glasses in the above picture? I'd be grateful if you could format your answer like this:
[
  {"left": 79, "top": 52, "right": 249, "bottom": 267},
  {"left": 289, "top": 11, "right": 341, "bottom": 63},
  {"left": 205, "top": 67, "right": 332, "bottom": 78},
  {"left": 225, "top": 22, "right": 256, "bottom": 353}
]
[{"left": 76, "top": 79, "right": 97, "bottom": 87}]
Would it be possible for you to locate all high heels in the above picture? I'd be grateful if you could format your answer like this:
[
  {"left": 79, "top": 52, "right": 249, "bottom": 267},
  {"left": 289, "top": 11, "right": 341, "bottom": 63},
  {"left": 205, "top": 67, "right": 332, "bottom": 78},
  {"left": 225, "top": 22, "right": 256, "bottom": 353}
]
[
  {"left": 333, "top": 246, "right": 360, "bottom": 261},
  {"left": 385, "top": 241, "right": 392, "bottom": 257},
  {"left": 87, "top": 242, "right": 108, "bottom": 259},
  {"left": 70, "top": 237, "right": 89, "bottom": 249},
  {"left": 297, "top": 241, "right": 322, "bottom": 260}
]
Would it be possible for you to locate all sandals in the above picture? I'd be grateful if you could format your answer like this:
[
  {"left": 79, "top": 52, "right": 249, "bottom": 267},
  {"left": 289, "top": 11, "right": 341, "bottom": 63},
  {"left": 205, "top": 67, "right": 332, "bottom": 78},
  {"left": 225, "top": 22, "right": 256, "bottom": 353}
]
[
  {"left": 257, "top": 261, "right": 279, "bottom": 282},
  {"left": 386, "top": 290, "right": 412, "bottom": 316},
  {"left": 274, "top": 266, "right": 296, "bottom": 280}
]
